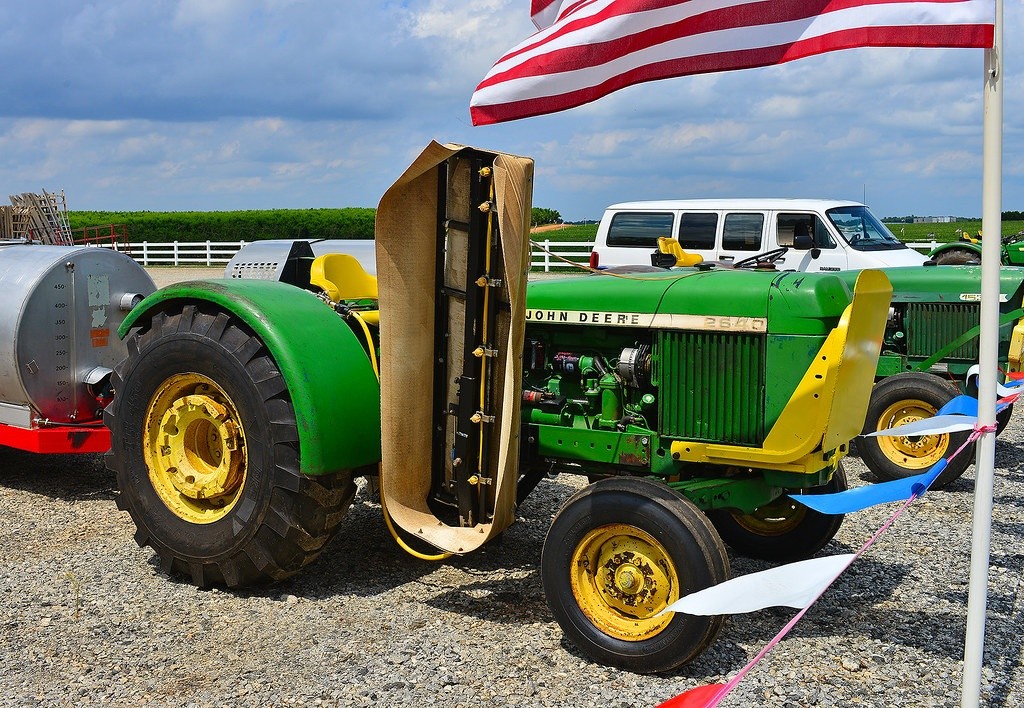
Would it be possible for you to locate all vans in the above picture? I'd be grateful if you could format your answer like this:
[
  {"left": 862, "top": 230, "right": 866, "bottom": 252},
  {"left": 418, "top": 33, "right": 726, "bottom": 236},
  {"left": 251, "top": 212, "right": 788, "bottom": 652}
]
[{"left": 591, "top": 195, "right": 928, "bottom": 272}]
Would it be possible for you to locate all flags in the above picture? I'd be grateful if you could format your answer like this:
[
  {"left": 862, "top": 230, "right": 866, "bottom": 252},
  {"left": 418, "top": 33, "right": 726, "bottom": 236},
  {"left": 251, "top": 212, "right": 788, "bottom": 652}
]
[{"left": 468, "top": 0, "right": 997, "bottom": 129}]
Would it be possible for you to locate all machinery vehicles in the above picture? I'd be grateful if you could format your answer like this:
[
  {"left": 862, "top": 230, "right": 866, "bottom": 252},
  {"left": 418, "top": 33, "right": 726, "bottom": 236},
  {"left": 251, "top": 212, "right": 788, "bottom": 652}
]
[
  {"left": 819, "top": 259, "right": 1024, "bottom": 486},
  {"left": 0, "top": 138, "right": 899, "bottom": 676}
]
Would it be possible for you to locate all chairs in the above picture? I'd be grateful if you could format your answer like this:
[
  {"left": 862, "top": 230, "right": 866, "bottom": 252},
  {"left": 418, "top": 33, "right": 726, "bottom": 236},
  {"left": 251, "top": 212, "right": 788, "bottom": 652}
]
[{"left": 794, "top": 221, "right": 819, "bottom": 248}]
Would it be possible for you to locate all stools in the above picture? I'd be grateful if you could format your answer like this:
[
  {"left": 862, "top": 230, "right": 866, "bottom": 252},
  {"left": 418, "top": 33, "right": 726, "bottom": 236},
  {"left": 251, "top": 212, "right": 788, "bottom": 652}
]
[
  {"left": 308, "top": 253, "right": 379, "bottom": 328},
  {"left": 963, "top": 232, "right": 978, "bottom": 243},
  {"left": 978, "top": 229, "right": 982, "bottom": 236},
  {"left": 658, "top": 236, "right": 704, "bottom": 267}
]
